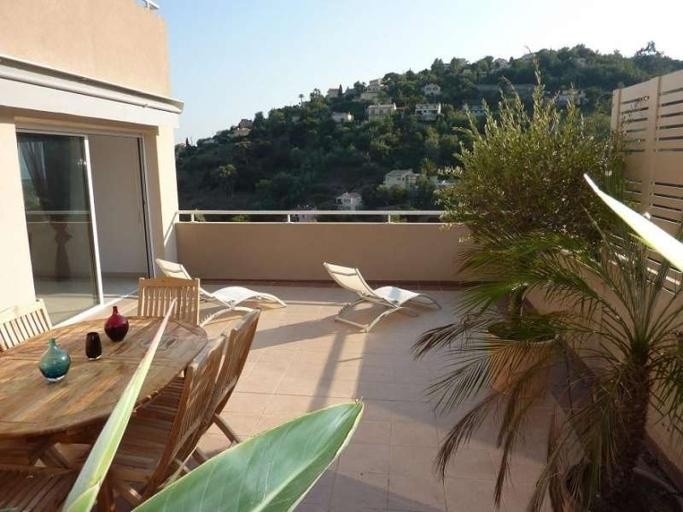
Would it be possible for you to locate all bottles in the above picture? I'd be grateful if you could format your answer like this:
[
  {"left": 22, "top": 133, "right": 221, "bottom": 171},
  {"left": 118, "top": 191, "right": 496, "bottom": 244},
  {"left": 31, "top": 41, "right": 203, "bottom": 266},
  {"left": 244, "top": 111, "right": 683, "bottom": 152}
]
[
  {"left": 103, "top": 306, "right": 128, "bottom": 341},
  {"left": 38, "top": 337, "right": 72, "bottom": 384},
  {"left": 85, "top": 332, "right": 103, "bottom": 361}
]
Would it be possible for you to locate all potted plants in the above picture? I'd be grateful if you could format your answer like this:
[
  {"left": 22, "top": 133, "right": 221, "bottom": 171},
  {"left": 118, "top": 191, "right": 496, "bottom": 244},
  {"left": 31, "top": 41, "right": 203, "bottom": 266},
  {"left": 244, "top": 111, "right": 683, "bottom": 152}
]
[{"left": 409, "top": 40, "right": 683, "bottom": 511}]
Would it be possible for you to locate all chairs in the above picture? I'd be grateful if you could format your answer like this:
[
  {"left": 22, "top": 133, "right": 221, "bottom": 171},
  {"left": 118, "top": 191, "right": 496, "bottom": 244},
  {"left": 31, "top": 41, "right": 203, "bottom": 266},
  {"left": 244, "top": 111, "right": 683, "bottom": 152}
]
[
  {"left": 155, "top": 259, "right": 287, "bottom": 328},
  {"left": 0, "top": 278, "right": 261, "bottom": 511},
  {"left": 322, "top": 260, "right": 441, "bottom": 334}
]
